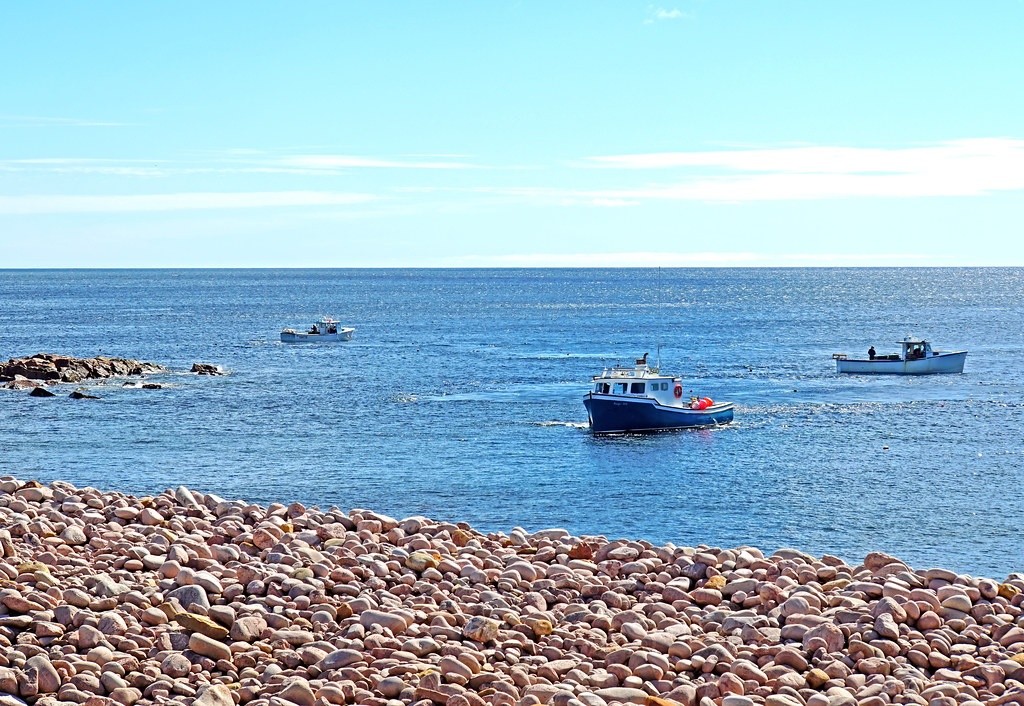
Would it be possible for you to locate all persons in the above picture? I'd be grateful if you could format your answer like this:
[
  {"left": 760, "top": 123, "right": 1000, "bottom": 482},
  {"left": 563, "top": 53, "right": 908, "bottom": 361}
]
[{"left": 868, "top": 345, "right": 877, "bottom": 360}]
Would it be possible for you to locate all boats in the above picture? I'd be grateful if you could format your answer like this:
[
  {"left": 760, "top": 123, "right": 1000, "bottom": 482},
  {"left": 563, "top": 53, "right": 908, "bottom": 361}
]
[
  {"left": 582, "top": 351, "right": 734, "bottom": 433},
  {"left": 281, "top": 317, "right": 356, "bottom": 342},
  {"left": 831, "top": 335, "right": 969, "bottom": 375}
]
